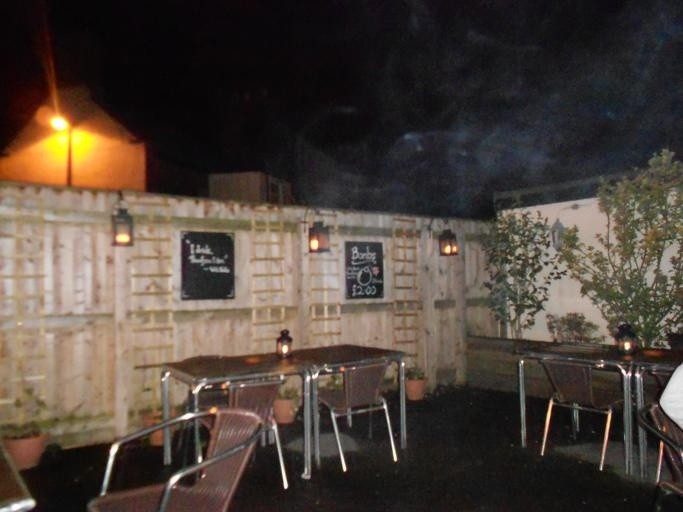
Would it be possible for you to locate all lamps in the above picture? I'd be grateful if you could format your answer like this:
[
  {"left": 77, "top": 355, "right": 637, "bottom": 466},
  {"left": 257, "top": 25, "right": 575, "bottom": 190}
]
[
  {"left": 616, "top": 324, "right": 637, "bottom": 357},
  {"left": 277, "top": 330, "right": 293, "bottom": 357},
  {"left": 303, "top": 204, "right": 332, "bottom": 253},
  {"left": 428, "top": 214, "right": 458, "bottom": 255},
  {"left": 112, "top": 191, "right": 134, "bottom": 247}
]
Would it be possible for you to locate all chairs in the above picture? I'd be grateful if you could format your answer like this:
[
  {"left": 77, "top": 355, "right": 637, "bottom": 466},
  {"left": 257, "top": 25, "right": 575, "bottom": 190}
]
[
  {"left": 87, "top": 407, "right": 265, "bottom": 512},
  {"left": 636, "top": 403, "right": 683, "bottom": 511}
]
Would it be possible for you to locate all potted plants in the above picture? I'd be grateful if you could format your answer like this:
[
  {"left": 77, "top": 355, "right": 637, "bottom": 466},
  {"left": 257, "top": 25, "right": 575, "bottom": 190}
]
[
  {"left": 406, "top": 367, "right": 429, "bottom": 401},
  {"left": 0, "top": 420, "right": 48, "bottom": 471}
]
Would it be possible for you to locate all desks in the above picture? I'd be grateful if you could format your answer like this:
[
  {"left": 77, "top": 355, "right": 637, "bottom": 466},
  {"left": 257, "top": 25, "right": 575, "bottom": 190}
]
[
  {"left": 161, "top": 345, "right": 407, "bottom": 475},
  {"left": 515, "top": 340, "right": 683, "bottom": 478},
  {"left": 0, "top": 440, "right": 39, "bottom": 512}
]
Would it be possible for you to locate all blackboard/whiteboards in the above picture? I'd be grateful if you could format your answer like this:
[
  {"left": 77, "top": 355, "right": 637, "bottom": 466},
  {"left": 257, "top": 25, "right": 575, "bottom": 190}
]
[
  {"left": 344, "top": 242, "right": 382, "bottom": 297},
  {"left": 180, "top": 233, "right": 235, "bottom": 299}
]
